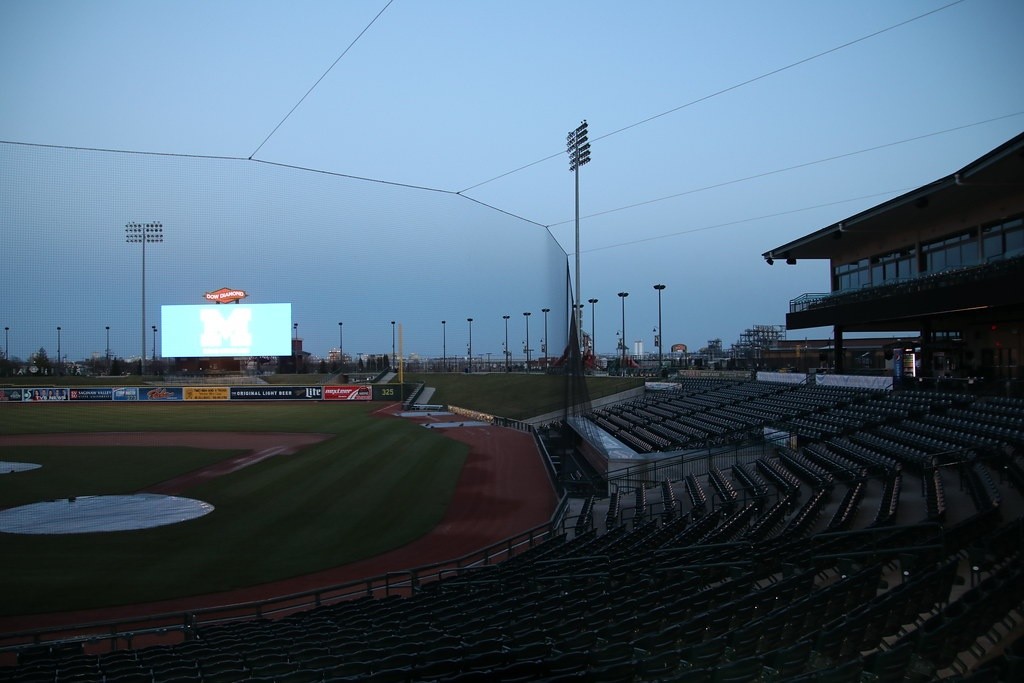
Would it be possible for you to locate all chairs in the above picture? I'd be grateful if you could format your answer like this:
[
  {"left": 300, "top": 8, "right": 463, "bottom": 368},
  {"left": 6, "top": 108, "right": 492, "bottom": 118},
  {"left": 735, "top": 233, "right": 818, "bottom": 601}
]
[{"left": 0, "top": 375, "right": 1024, "bottom": 683}]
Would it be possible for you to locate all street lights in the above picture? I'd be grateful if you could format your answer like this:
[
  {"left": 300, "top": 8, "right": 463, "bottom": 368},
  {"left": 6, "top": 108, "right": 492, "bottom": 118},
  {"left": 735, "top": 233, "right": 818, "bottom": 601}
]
[
  {"left": 467, "top": 318, "right": 473, "bottom": 367},
  {"left": 615, "top": 291, "right": 629, "bottom": 377},
  {"left": 441, "top": 320, "right": 446, "bottom": 371},
  {"left": 4, "top": 327, "right": 9, "bottom": 360},
  {"left": 653, "top": 283, "right": 666, "bottom": 369},
  {"left": 523, "top": 312, "right": 531, "bottom": 373},
  {"left": 152, "top": 325, "right": 157, "bottom": 359},
  {"left": 566, "top": 119, "right": 591, "bottom": 355},
  {"left": 338, "top": 321, "right": 344, "bottom": 363},
  {"left": 293, "top": 322, "right": 298, "bottom": 338},
  {"left": 57, "top": 326, "right": 62, "bottom": 362},
  {"left": 588, "top": 298, "right": 598, "bottom": 356},
  {"left": 573, "top": 303, "right": 584, "bottom": 349},
  {"left": 391, "top": 321, "right": 395, "bottom": 372},
  {"left": 123, "top": 220, "right": 166, "bottom": 377},
  {"left": 106, "top": 326, "right": 110, "bottom": 359},
  {"left": 541, "top": 307, "right": 550, "bottom": 370},
  {"left": 502, "top": 314, "right": 510, "bottom": 372}
]
